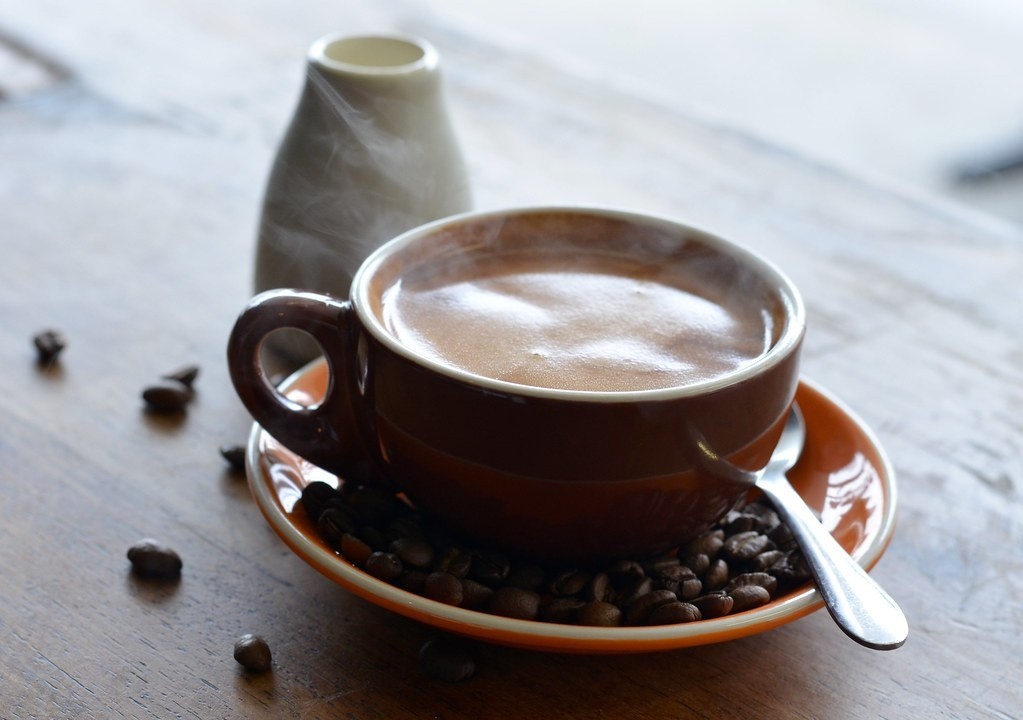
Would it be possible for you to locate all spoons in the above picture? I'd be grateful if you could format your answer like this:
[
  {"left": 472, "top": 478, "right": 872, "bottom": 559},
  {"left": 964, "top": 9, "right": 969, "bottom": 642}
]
[{"left": 740, "top": 401, "right": 908, "bottom": 650}]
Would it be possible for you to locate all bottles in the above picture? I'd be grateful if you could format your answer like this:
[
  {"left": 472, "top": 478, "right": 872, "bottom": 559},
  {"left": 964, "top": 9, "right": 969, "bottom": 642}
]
[{"left": 251, "top": 29, "right": 478, "bottom": 364}]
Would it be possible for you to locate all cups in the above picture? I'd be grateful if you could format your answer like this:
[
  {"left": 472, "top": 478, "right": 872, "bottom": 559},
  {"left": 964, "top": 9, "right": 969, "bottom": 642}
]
[{"left": 229, "top": 206, "right": 807, "bottom": 561}]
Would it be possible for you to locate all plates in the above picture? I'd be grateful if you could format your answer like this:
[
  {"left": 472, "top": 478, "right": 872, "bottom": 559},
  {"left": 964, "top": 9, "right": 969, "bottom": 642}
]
[{"left": 248, "top": 357, "right": 898, "bottom": 649}]
